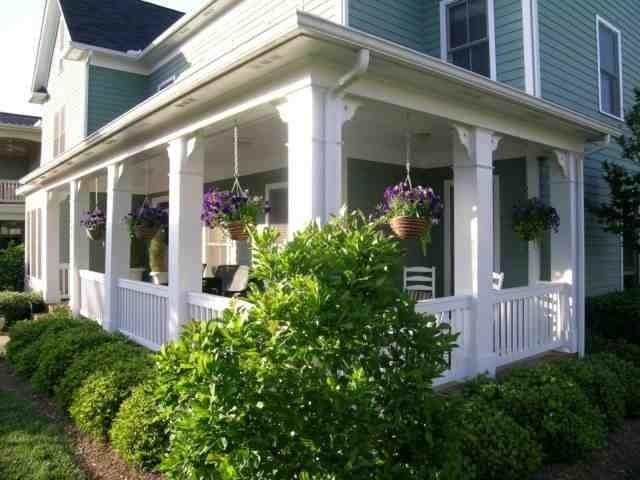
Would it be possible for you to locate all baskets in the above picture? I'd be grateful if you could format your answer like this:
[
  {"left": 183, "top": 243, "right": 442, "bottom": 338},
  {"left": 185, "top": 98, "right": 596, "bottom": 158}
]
[
  {"left": 219, "top": 218, "right": 256, "bottom": 241},
  {"left": 86, "top": 227, "right": 105, "bottom": 240},
  {"left": 129, "top": 222, "right": 162, "bottom": 241}
]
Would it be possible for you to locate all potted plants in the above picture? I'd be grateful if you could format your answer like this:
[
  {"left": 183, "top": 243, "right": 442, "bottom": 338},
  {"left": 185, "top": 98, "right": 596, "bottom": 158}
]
[{"left": 148, "top": 231, "right": 167, "bottom": 283}]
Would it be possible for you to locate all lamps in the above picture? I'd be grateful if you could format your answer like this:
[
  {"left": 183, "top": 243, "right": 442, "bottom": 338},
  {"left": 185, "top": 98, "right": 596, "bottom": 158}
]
[
  {"left": 28, "top": 86, "right": 48, "bottom": 104},
  {"left": 63, "top": 47, "right": 88, "bottom": 61}
]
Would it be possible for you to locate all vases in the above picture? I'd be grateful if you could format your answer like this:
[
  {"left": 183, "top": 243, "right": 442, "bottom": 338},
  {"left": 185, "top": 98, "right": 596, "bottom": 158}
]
[
  {"left": 226, "top": 221, "right": 255, "bottom": 240},
  {"left": 514, "top": 223, "right": 543, "bottom": 239},
  {"left": 86, "top": 225, "right": 104, "bottom": 240},
  {"left": 134, "top": 226, "right": 156, "bottom": 240}
]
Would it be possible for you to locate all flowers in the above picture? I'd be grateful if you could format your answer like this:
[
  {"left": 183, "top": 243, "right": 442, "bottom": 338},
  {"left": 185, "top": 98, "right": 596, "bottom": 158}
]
[
  {"left": 199, "top": 186, "right": 271, "bottom": 229},
  {"left": 79, "top": 207, "right": 106, "bottom": 225},
  {"left": 378, "top": 182, "right": 443, "bottom": 226},
  {"left": 125, "top": 206, "right": 168, "bottom": 228},
  {"left": 510, "top": 197, "right": 560, "bottom": 247}
]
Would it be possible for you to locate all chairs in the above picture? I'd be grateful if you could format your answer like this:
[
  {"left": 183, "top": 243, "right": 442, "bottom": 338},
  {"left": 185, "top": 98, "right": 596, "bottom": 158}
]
[
  {"left": 493, "top": 271, "right": 504, "bottom": 290},
  {"left": 401, "top": 266, "right": 436, "bottom": 302},
  {"left": 203, "top": 263, "right": 251, "bottom": 298}
]
